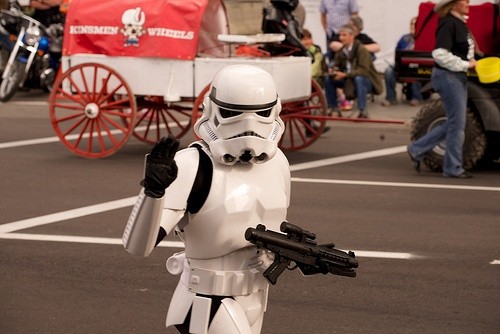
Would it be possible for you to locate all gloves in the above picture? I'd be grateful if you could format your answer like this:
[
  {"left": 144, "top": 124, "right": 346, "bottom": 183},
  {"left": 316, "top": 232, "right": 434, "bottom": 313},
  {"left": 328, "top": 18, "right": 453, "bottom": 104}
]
[
  {"left": 299, "top": 243, "right": 335, "bottom": 275},
  {"left": 146, "top": 135, "right": 179, "bottom": 196}
]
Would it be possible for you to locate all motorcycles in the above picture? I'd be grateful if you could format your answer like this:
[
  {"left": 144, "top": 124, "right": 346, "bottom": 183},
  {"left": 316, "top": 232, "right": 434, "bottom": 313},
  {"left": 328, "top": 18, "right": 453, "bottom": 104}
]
[{"left": 0, "top": 0, "right": 64, "bottom": 103}]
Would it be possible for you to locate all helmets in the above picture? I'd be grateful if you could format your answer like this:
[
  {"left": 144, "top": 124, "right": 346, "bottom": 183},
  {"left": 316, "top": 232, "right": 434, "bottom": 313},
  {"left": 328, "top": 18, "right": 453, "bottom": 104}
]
[{"left": 194, "top": 64, "right": 285, "bottom": 164}]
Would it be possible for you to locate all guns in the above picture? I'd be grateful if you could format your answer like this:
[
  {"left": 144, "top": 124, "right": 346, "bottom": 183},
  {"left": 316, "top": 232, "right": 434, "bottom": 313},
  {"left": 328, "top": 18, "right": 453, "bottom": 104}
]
[{"left": 243, "top": 221, "right": 359, "bottom": 284}]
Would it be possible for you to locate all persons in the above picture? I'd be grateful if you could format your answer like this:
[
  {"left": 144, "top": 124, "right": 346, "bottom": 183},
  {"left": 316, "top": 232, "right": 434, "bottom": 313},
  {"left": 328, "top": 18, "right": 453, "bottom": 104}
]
[
  {"left": 407, "top": 0, "right": 479, "bottom": 179},
  {"left": 320, "top": 0, "right": 359, "bottom": 53},
  {"left": 382, "top": 16, "right": 423, "bottom": 105},
  {"left": 121, "top": 64, "right": 292, "bottom": 334},
  {"left": 300, "top": 31, "right": 324, "bottom": 92},
  {"left": 29, "top": 0, "right": 68, "bottom": 93},
  {"left": 324, "top": 24, "right": 384, "bottom": 119},
  {"left": 328, "top": 16, "right": 380, "bottom": 74}
]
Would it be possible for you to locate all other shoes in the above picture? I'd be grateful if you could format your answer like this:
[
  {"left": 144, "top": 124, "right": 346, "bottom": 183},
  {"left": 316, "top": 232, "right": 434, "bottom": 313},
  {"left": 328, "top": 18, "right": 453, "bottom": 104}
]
[
  {"left": 327, "top": 109, "right": 342, "bottom": 117},
  {"left": 453, "top": 171, "right": 472, "bottom": 178},
  {"left": 407, "top": 149, "right": 421, "bottom": 174},
  {"left": 305, "top": 126, "right": 329, "bottom": 138},
  {"left": 358, "top": 110, "right": 369, "bottom": 118}
]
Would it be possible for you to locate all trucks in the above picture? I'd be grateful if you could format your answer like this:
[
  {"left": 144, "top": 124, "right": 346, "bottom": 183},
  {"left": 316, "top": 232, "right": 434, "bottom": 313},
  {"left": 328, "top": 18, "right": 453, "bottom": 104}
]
[{"left": 396, "top": 0, "right": 500, "bottom": 172}]
